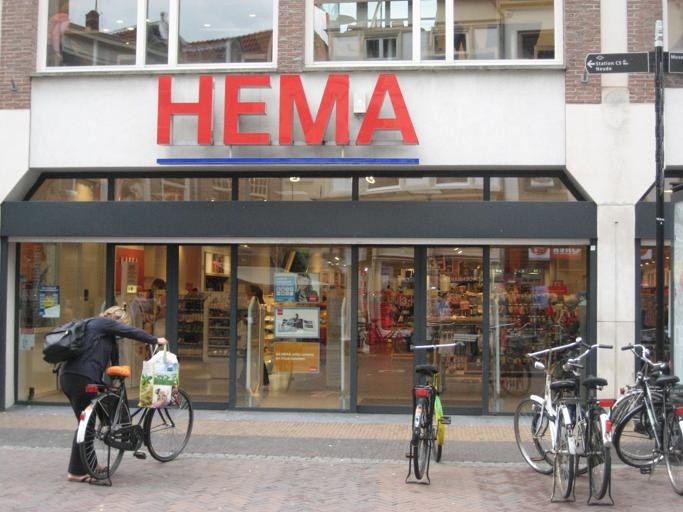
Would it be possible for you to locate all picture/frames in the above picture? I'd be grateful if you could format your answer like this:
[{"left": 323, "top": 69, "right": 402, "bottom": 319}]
[
  {"left": 274, "top": 342, "right": 320, "bottom": 373},
  {"left": 274, "top": 307, "right": 320, "bottom": 339},
  {"left": 204, "top": 249, "right": 231, "bottom": 275}
]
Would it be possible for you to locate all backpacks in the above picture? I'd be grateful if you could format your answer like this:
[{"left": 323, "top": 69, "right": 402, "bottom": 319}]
[{"left": 42, "top": 318, "right": 88, "bottom": 363}]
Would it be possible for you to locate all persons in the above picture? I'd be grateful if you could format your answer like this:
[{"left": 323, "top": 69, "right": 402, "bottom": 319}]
[
  {"left": 59, "top": 306, "right": 168, "bottom": 484},
  {"left": 237, "top": 283, "right": 269, "bottom": 386},
  {"left": 151, "top": 277, "right": 167, "bottom": 342},
  {"left": 295, "top": 273, "right": 312, "bottom": 301},
  {"left": 46, "top": 0, "right": 81, "bottom": 65}
]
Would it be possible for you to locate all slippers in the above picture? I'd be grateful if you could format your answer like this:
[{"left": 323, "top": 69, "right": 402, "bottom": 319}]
[{"left": 69, "top": 476, "right": 97, "bottom": 482}]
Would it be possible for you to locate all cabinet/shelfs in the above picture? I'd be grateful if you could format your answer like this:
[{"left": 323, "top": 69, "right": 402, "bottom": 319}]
[
  {"left": 394, "top": 277, "right": 559, "bottom": 369},
  {"left": 177, "top": 293, "right": 211, "bottom": 361},
  {"left": 203, "top": 294, "right": 251, "bottom": 363}
]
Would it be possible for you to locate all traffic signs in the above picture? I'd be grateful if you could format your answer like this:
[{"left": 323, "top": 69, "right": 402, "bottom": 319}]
[
  {"left": 669, "top": 51, "right": 683, "bottom": 73},
  {"left": 584, "top": 53, "right": 651, "bottom": 74}
]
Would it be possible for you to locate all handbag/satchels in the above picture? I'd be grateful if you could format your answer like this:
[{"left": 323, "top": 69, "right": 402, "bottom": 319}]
[{"left": 139, "top": 344, "right": 180, "bottom": 409}]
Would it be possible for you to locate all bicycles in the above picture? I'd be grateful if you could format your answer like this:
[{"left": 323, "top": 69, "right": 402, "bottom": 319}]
[
  {"left": 357, "top": 308, "right": 412, "bottom": 355},
  {"left": 403, "top": 339, "right": 469, "bottom": 488},
  {"left": 510, "top": 334, "right": 683, "bottom": 508},
  {"left": 73, "top": 340, "right": 197, "bottom": 487},
  {"left": 492, "top": 298, "right": 585, "bottom": 400}
]
